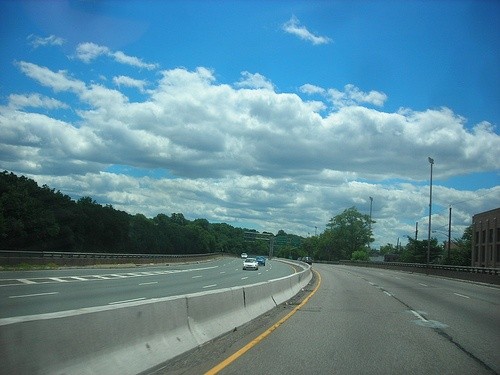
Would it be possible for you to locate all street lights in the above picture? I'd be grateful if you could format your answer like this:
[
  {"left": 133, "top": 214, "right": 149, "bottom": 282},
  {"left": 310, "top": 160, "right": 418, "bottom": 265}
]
[
  {"left": 366, "top": 195, "right": 373, "bottom": 264},
  {"left": 425, "top": 157, "right": 434, "bottom": 275}
]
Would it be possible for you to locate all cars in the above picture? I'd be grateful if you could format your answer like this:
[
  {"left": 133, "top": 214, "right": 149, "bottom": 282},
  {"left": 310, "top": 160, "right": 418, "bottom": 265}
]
[
  {"left": 256, "top": 256, "right": 266, "bottom": 266},
  {"left": 302, "top": 257, "right": 312, "bottom": 264},
  {"left": 243, "top": 258, "right": 258, "bottom": 271},
  {"left": 241, "top": 252, "right": 248, "bottom": 259}
]
